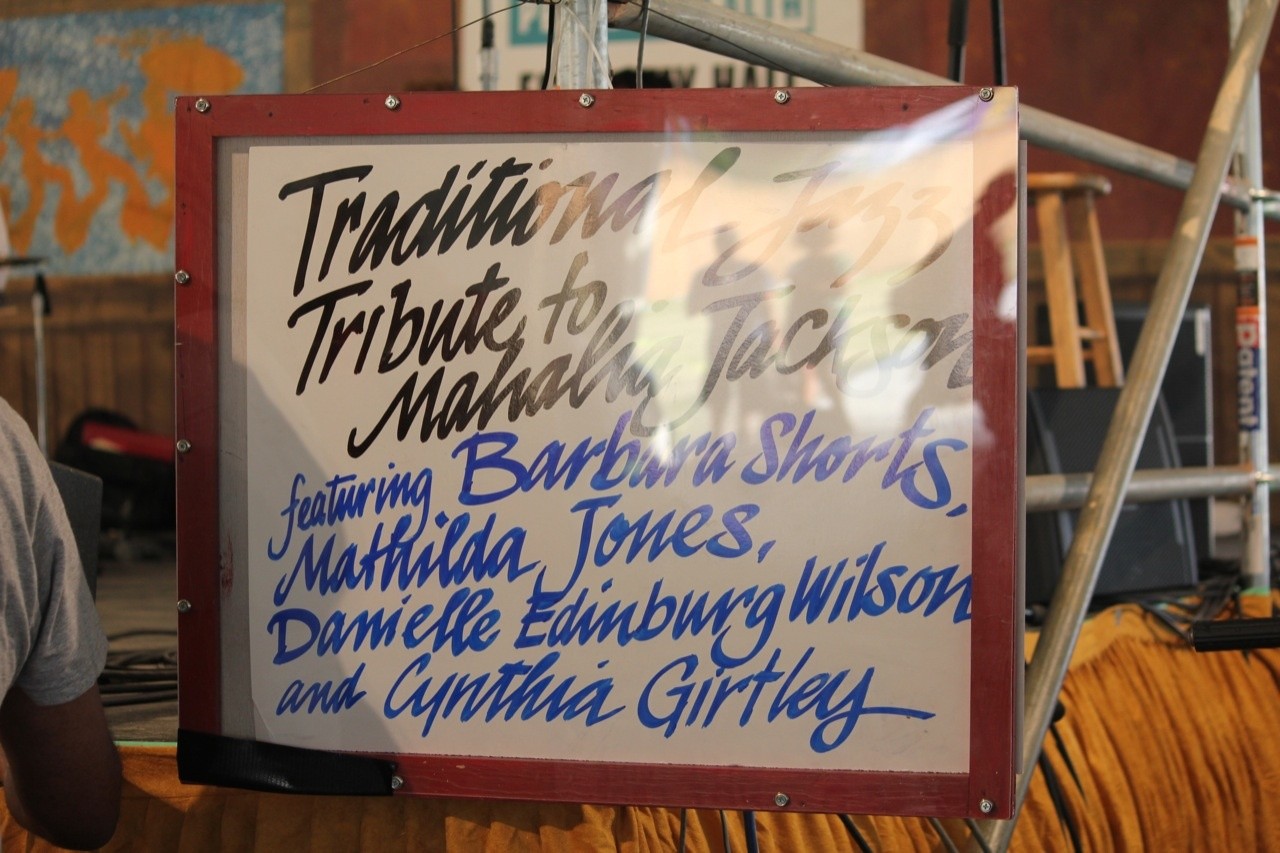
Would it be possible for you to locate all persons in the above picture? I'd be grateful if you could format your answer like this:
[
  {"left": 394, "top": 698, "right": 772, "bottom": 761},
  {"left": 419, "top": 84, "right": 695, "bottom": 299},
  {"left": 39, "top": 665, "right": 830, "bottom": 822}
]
[{"left": 0, "top": 397, "right": 126, "bottom": 853}]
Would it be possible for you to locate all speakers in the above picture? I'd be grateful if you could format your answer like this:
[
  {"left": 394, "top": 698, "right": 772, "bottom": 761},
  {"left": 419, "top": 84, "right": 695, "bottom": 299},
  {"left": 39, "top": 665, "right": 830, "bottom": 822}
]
[{"left": 1022, "top": 387, "right": 1198, "bottom": 607}]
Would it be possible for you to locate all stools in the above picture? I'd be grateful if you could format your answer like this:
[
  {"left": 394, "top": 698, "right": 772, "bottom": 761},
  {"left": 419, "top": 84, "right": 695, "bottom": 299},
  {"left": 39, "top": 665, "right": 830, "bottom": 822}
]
[{"left": 1021, "top": 172, "right": 1126, "bottom": 392}]
